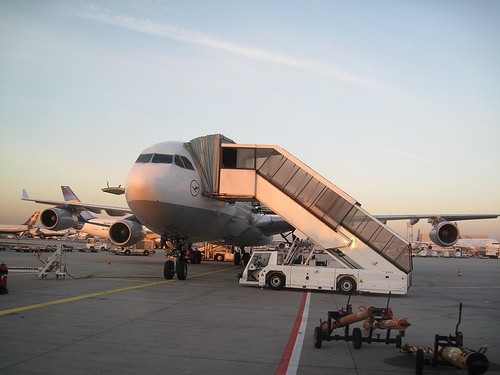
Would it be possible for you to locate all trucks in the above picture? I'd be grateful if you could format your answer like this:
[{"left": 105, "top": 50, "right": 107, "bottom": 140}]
[
  {"left": 238, "top": 145, "right": 413, "bottom": 296},
  {"left": 135, "top": 239, "right": 156, "bottom": 254}
]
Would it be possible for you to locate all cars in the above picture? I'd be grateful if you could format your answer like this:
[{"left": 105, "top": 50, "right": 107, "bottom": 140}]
[{"left": 114, "top": 245, "right": 150, "bottom": 256}]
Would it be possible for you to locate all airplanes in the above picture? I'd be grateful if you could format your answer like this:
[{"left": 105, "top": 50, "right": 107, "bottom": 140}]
[
  {"left": 19, "top": 140, "right": 500, "bottom": 281},
  {"left": 0, "top": 210, "right": 76, "bottom": 241},
  {"left": 410, "top": 238, "right": 500, "bottom": 259}
]
